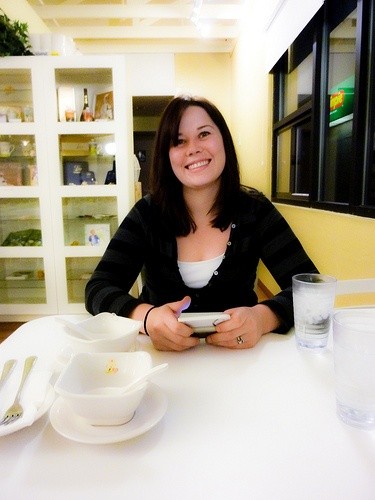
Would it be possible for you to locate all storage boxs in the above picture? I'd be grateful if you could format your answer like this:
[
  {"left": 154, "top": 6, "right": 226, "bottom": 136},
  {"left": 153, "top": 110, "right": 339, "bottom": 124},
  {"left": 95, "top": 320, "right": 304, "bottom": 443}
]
[{"left": 57, "top": 87, "right": 77, "bottom": 123}]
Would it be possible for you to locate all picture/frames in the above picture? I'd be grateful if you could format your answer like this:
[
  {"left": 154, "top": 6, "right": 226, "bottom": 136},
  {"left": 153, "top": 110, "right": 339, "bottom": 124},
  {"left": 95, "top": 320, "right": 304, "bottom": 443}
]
[{"left": 0, "top": 163, "right": 22, "bottom": 186}]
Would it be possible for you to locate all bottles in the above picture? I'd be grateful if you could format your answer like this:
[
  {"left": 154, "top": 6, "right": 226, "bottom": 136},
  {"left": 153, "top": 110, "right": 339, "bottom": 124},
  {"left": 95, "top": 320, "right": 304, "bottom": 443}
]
[
  {"left": 88, "top": 138, "right": 98, "bottom": 157},
  {"left": 80, "top": 89, "right": 93, "bottom": 121}
]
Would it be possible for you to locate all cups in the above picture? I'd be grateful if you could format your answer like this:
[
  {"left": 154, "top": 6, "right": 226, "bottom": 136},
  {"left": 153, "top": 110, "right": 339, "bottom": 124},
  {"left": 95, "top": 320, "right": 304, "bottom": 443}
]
[
  {"left": 291, "top": 273, "right": 337, "bottom": 347},
  {"left": 332, "top": 305, "right": 375, "bottom": 428}
]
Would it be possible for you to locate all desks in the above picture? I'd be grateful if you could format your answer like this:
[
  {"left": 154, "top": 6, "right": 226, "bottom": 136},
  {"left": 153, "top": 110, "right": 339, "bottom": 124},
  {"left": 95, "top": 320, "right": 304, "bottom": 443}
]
[{"left": 0, "top": 314, "right": 375, "bottom": 499}]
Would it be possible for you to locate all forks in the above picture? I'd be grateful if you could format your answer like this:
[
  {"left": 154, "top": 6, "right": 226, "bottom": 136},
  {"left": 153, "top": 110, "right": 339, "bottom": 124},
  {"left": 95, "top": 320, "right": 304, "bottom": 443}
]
[{"left": 1, "top": 355, "right": 38, "bottom": 426}]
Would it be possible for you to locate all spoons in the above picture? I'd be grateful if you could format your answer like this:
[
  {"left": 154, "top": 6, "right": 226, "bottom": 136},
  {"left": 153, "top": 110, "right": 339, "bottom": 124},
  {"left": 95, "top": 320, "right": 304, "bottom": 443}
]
[{"left": 93, "top": 361, "right": 168, "bottom": 396}]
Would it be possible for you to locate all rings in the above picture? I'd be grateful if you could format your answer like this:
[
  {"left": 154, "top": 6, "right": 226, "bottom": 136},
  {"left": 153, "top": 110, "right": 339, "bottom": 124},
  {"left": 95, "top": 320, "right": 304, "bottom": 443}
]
[{"left": 236, "top": 336, "right": 244, "bottom": 344}]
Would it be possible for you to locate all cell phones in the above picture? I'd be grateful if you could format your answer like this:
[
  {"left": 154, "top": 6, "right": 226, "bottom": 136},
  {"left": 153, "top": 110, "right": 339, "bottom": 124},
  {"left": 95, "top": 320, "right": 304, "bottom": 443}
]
[{"left": 178, "top": 315, "right": 232, "bottom": 328}]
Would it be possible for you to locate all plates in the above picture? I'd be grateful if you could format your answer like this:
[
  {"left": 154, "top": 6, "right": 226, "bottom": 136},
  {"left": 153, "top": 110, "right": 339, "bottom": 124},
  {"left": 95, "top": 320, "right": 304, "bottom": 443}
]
[{"left": 1, "top": 384, "right": 54, "bottom": 439}]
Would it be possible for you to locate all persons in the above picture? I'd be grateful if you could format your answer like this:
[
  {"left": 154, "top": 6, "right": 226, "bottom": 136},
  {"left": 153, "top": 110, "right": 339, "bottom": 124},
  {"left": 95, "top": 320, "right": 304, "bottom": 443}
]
[{"left": 85, "top": 94, "right": 324, "bottom": 352}]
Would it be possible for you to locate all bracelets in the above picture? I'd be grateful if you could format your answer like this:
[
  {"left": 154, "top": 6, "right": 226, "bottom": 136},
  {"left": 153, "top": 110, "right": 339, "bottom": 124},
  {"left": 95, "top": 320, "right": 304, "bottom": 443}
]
[{"left": 145, "top": 307, "right": 158, "bottom": 336}]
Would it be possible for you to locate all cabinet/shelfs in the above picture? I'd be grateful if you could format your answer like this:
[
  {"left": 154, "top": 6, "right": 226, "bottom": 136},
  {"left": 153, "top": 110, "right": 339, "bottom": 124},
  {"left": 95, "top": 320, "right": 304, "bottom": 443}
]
[{"left": 0, "top": 56, "right": 138, "bottom": 322}]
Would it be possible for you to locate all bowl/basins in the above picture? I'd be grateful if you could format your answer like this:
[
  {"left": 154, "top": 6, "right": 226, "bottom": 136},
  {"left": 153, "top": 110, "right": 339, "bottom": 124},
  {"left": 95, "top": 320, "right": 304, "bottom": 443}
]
[
  {"left": 58, "top": 350, "right": 153, "bottom": 426},
  {"left": 67, "top": 312, "right": 143, "bottom": 363}
]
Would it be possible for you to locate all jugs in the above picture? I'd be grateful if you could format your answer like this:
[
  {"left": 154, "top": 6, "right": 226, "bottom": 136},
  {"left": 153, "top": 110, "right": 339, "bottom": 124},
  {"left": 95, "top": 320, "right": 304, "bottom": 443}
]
[{"left": 0, "top": 142, "right": 15, "bottom": 157}]
